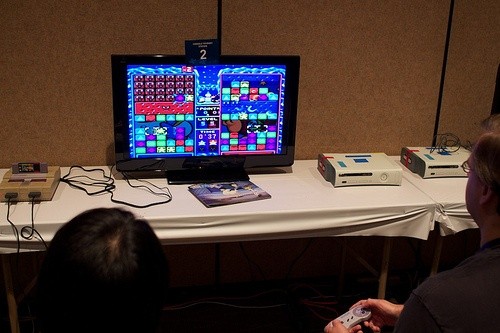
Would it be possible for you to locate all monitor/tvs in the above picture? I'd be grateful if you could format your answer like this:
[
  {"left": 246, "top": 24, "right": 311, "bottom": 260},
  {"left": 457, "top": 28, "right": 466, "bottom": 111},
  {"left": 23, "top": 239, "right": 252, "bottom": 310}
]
[{"left": 110, "top": 53, "right": 299, "bottom": 185}]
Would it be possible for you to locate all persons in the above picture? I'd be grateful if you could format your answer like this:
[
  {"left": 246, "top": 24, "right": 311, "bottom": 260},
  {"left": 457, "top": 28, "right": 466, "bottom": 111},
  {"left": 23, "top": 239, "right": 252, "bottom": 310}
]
[
  {"left": 40, "top": 207, "right": 167, "bottom": 333},
  {"left": 324, "top": 115, "right": 500, "bottom": 333}
]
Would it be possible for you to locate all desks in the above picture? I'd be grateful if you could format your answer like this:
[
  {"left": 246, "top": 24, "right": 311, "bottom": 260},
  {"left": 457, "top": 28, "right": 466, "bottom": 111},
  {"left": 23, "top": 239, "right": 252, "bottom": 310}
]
[
  {"left": 388, "top": 156, "right": 480, "bottom": 278},
  {"left": 0, "top": 160, "right": 438, "bottom": 333}
]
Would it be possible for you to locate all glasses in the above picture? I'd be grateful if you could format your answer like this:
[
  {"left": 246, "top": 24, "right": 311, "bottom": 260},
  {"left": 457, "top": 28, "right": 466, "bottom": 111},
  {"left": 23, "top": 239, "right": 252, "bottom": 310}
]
[{"left": 461, "top": 161, "right": 475, "bottom": 173}]
